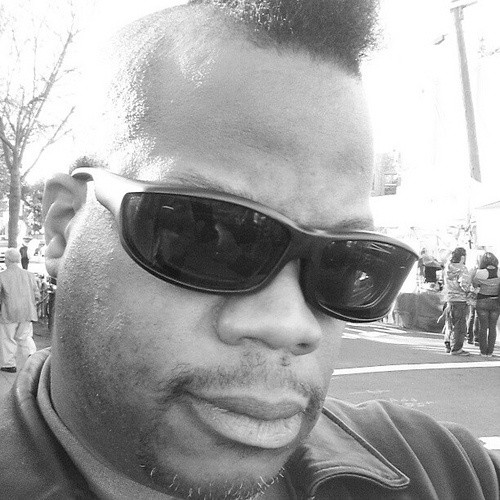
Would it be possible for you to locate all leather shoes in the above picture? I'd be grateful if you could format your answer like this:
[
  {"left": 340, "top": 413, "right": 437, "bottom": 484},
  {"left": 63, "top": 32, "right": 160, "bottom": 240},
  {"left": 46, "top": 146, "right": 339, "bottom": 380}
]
[{"left": 2, "top": 366, "right": 16, "bottom": 373}]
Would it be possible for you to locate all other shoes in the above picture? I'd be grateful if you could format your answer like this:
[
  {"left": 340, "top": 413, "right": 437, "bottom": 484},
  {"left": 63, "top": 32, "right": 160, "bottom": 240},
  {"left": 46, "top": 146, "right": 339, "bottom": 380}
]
[{"left": 446, "top": 340, "right": 493, "bottom": 357}]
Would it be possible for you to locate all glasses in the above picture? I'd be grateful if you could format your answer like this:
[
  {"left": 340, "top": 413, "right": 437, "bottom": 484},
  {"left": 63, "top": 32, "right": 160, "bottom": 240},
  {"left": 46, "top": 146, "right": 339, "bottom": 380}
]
[{"left": 70, "top": 166, "right": 418, "bottom": 323}]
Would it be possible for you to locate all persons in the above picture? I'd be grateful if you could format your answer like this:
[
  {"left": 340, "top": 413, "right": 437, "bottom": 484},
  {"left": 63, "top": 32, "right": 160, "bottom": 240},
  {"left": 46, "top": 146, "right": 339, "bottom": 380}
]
[
  {"left": 443, "top": 246, "right": 500, "bottom": 359},
  {"left": 0, "top": 0, "right": 500, "bottom": 500},
  {"left": 0, "top": 247, "right": 39, "bottom": 373}
]
